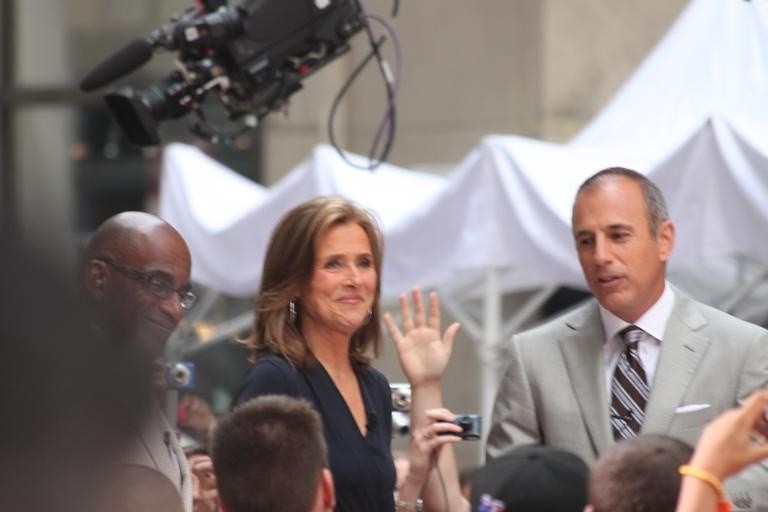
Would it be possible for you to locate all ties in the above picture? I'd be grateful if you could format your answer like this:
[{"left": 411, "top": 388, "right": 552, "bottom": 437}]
[{"left": 612, "top": 325, "right": 650, "bottom": 443}]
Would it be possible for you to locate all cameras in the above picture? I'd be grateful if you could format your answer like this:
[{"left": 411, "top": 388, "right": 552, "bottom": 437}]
[
  {"left": 391, "top": 413, "right": 410, "bottom": 435},
  {"left": 390, "top": 382, "right": 412, "bottom": 412},
  {"left": 161, "top": 362, "right": 197, "bottom": 391},
  {"left": 437, "top": 414, "right": 482, "bottom": 440}
]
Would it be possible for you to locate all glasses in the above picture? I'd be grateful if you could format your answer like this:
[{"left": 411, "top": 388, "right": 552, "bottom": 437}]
[{"left": 93, "top": 256, "right": 197, "bottom": 312}]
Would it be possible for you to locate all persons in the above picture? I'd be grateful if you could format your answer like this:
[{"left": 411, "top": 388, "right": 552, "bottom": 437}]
[{"left": 82, "top": 166, "right": 767, "bottom": 512}]
